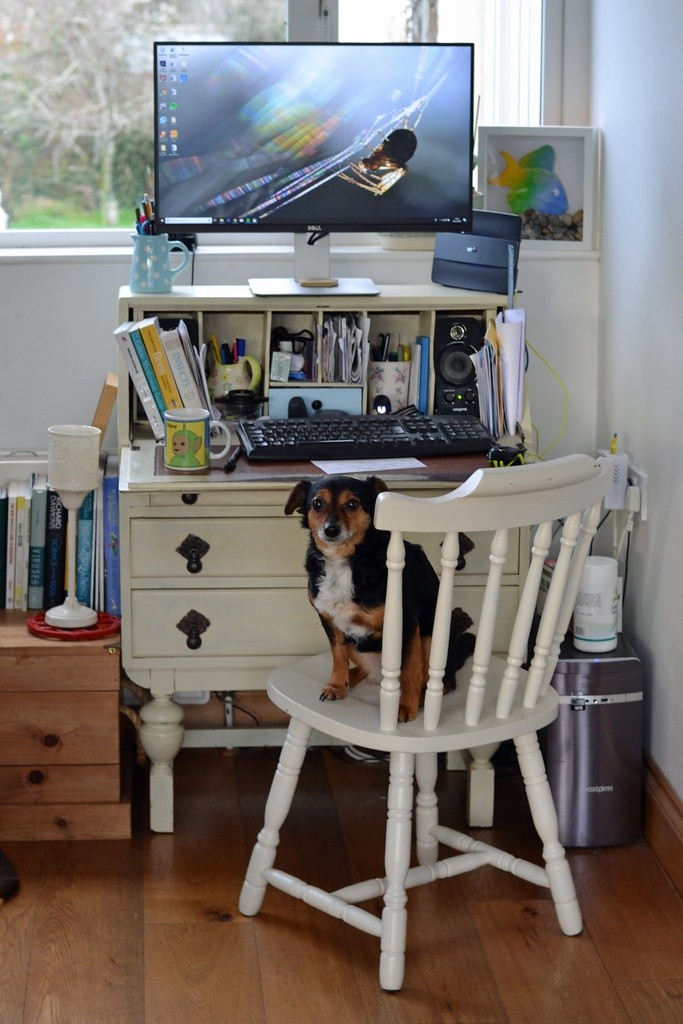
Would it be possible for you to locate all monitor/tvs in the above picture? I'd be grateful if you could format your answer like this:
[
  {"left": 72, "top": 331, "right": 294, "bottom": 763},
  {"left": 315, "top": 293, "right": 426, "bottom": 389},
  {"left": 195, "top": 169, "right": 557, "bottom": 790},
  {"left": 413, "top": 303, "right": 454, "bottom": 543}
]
[{"left": 151, "top": 41, "right": 475, "bottom": 233}]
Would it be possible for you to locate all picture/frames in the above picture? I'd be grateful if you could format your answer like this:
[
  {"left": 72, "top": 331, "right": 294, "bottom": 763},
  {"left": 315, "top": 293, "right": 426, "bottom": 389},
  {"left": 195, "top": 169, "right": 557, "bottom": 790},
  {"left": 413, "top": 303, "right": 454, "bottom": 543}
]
[{"left": 477, "top": 126, "right": 601, "bottom": 251}]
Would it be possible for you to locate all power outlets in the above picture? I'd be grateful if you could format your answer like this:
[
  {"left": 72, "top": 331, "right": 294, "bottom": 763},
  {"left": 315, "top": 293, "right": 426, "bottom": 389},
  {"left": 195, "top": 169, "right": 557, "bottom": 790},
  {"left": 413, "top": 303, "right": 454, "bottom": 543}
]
[{"left": 626, "top": 465, "right": 648, "bottom": 522}]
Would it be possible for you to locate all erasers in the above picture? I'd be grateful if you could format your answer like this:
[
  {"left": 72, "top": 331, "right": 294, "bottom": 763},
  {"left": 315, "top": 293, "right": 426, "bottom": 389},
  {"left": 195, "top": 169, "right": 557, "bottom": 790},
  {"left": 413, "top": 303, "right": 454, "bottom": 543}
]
[{"left": 299, "top": 277, "right": 339, "bottom": 287}]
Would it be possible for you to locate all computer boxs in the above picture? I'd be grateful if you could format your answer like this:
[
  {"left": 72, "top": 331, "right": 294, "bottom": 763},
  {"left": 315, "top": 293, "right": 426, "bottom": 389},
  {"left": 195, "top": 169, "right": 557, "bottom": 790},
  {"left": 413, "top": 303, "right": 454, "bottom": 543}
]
[{"left": 549, "top": 630, "right": 643, "bottom": 847}]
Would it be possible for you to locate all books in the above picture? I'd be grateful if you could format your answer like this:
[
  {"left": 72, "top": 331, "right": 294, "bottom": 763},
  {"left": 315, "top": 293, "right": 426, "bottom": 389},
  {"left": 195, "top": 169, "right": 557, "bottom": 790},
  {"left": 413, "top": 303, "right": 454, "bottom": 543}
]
[
  {"left": 112, "top": 316, "right": 224, "bottom": 440},
  {"left": 0, "top": 453, "right": 120, "bottom": 615},
  {"left": 408, "top": 334, "right": 429, "bottom": 416}
]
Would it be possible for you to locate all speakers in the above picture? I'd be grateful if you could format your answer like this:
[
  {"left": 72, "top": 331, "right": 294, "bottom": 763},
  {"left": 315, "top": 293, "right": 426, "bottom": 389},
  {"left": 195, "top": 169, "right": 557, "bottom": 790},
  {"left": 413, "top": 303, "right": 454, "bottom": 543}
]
[{"left": 435, "top": 315, "right": 484, "bottom": 417}]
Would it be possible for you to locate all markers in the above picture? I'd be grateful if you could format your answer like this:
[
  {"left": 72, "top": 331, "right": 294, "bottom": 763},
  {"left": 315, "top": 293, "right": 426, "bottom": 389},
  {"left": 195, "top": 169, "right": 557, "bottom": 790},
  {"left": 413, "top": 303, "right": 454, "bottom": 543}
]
[
  {"left": 223, "top": 446, "right": 241, "bottom": 473},
  {"left": 369, "top": 331, "right": 411, "bottom": 361}
]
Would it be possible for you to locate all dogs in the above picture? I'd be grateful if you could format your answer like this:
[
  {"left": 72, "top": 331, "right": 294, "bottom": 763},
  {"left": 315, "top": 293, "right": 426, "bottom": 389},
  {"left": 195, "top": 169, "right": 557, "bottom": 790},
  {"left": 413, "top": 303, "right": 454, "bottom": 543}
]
[{"left": 284, "top": 475, "right": 476, "bottom": 724}]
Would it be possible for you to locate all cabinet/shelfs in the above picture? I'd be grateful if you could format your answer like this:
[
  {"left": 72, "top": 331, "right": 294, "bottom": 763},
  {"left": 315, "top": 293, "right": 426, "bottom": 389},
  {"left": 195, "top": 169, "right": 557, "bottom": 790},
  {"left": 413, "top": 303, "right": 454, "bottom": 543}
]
[
  {"left": 1, "top": 605, "right": 133, "bottom": 842},
  {"left": 117, "top": 280, "right": 533, "bottom": 836}
]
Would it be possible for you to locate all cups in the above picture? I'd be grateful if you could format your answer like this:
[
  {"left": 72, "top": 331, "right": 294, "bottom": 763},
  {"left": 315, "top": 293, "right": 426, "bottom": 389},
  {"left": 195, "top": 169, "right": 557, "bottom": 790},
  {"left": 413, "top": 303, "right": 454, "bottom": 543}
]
[
  {"left": 208, "top": 348, "right": 262, "bottom": 422},
  {"left": 163, "top": 408, "right": 230, "bottom": 472},
  {"left": 128, "top": 233, "right": 190, "bottom": 294},
  {"left": 368, "top": 361, "right": 410, "bottom": 418}
]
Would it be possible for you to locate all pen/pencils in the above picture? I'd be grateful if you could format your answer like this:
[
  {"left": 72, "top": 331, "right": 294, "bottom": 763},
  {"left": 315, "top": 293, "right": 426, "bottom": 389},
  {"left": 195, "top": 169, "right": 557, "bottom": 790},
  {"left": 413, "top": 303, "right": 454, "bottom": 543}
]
[
  {"left": 133, "top": 193, "right": 164, "bottom": 235},
  {"left": 207, "top": 333, "right": 240, "bottom": 364}
]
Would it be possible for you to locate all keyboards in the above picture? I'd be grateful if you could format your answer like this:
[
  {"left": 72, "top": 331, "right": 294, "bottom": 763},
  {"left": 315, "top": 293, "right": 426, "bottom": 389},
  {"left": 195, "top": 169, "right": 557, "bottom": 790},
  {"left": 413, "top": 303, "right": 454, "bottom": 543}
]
[{"left": 236, "top": 414, "right": 495, "bottom": 461}]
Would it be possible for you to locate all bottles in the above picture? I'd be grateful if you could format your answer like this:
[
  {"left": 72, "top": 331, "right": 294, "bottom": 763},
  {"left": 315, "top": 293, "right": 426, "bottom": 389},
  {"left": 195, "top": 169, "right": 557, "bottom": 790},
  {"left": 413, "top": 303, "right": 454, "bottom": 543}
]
[{"left": 572, "top": 556, "right": 618, "bottom": 654}]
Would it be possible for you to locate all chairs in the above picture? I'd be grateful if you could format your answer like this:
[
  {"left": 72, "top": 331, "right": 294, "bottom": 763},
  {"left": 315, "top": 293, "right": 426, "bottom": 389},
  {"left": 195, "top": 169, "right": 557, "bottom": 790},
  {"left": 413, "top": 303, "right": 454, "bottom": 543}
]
[{"left": 235, "top": 452, "right": 609, "bottom": 994}]
